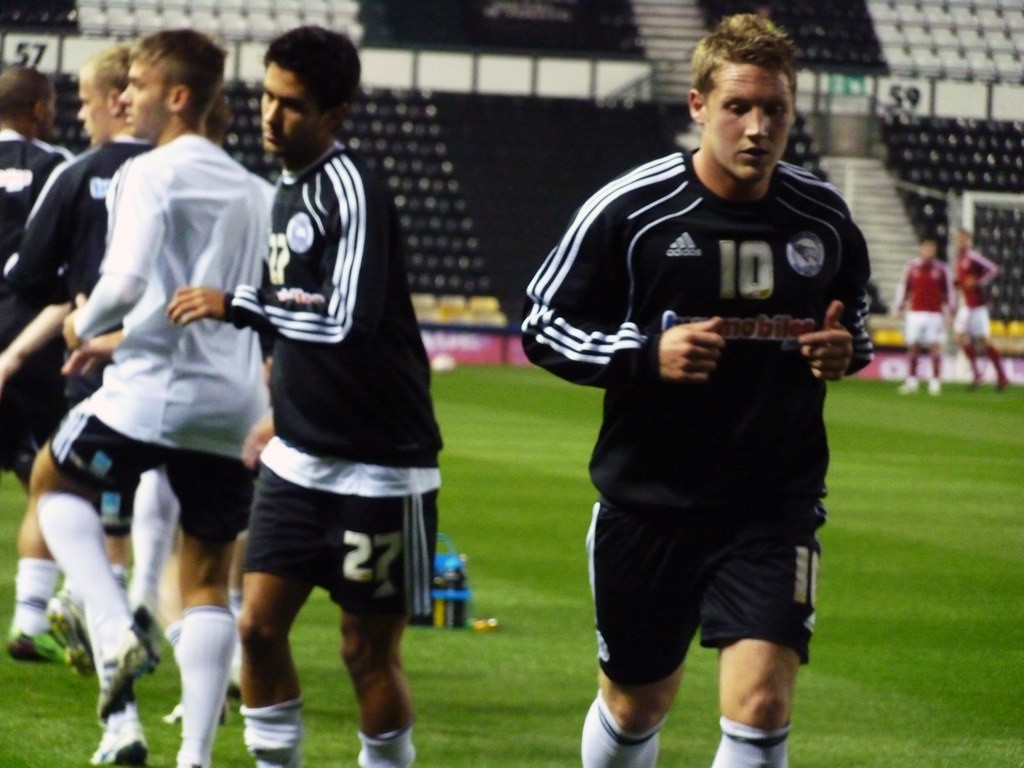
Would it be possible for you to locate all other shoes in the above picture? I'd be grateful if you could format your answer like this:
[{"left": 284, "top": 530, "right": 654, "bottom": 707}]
[
  {"left": 997, "top": 378, "right": 1009, "bottom": 393},
  {"left": 89, "top": 703, "right": 149, "bottom": 765},
  {"left": 46, "top": 588, "right": 97, "bottom": 677},
  {"left": 928, "top": 377, "right": 942, "bottom": 396},
  {"left": 9, "top": 608, "right": 41, "bottom": 660},
  {"left": 898, "top": 376, "right": 921, "bottom": 395},
  {"left": 98, "top": 627, "right": 159, "bottom": 721},
  {"left": 969, "top": 374, "right": 983, "bottom": 389}
]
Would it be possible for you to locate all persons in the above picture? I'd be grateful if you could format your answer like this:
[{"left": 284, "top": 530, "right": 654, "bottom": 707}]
[
  {"left": 521, "top": 15, "right": 874, "bottom": 768},
  {"left": 0, "top": 26, "right": 445, "bottom": 768},
  {"left": 893, "top": 223, "right": 1010, "bottom": 395}
]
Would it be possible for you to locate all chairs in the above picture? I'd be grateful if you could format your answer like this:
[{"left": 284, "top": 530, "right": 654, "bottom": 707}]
[{"left": 0, "top": 0, "right": 1024, "bottom": 359}]
